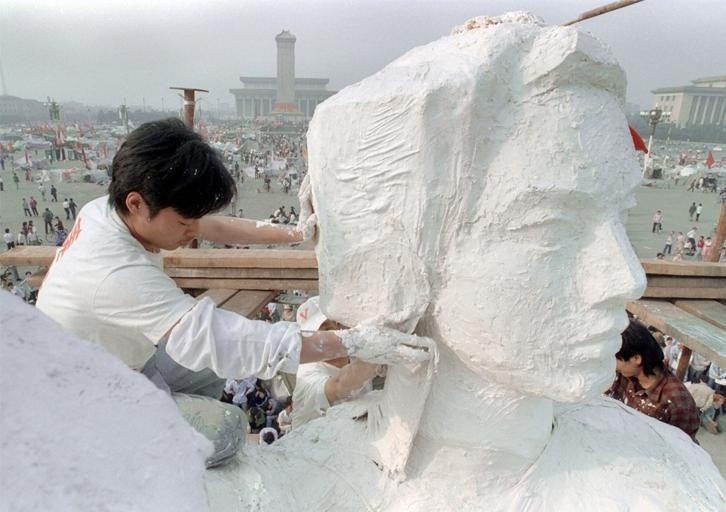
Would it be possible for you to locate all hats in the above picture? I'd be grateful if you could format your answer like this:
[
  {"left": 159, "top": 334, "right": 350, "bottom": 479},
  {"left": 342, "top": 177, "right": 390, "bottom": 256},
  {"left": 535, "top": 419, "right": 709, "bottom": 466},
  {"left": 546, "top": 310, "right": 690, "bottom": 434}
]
[{"left": 295, "top": 294, "right": 331, "bottom": 334}]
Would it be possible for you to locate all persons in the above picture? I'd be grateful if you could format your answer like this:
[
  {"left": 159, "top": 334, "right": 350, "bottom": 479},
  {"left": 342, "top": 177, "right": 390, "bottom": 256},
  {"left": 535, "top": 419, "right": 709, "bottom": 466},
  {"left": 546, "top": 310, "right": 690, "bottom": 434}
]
[
  {"left": 289, "top": 293, "right": 380, "bottom": 434},
  {"left": 215, "top": 10, "right": 724, "bottom": 512},
  {"left": 603, "top": 315, "right": 700, "bottom": 445},
  {"left": 626, "top": 142, "right": 725, "bottom": 435},
  {"left": 34, "top": 116, "right": 439, "bottom": 473}
]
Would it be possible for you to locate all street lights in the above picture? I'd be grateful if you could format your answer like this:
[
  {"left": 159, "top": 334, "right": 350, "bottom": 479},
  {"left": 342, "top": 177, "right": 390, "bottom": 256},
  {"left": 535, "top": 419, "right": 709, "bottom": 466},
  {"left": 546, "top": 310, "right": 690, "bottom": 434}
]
[
  {"left": 43, "top": 98, "right": 62, "bottom": 122},
  {"left": 640, "top": 103, "right": 671, "bottom": 177}
]
